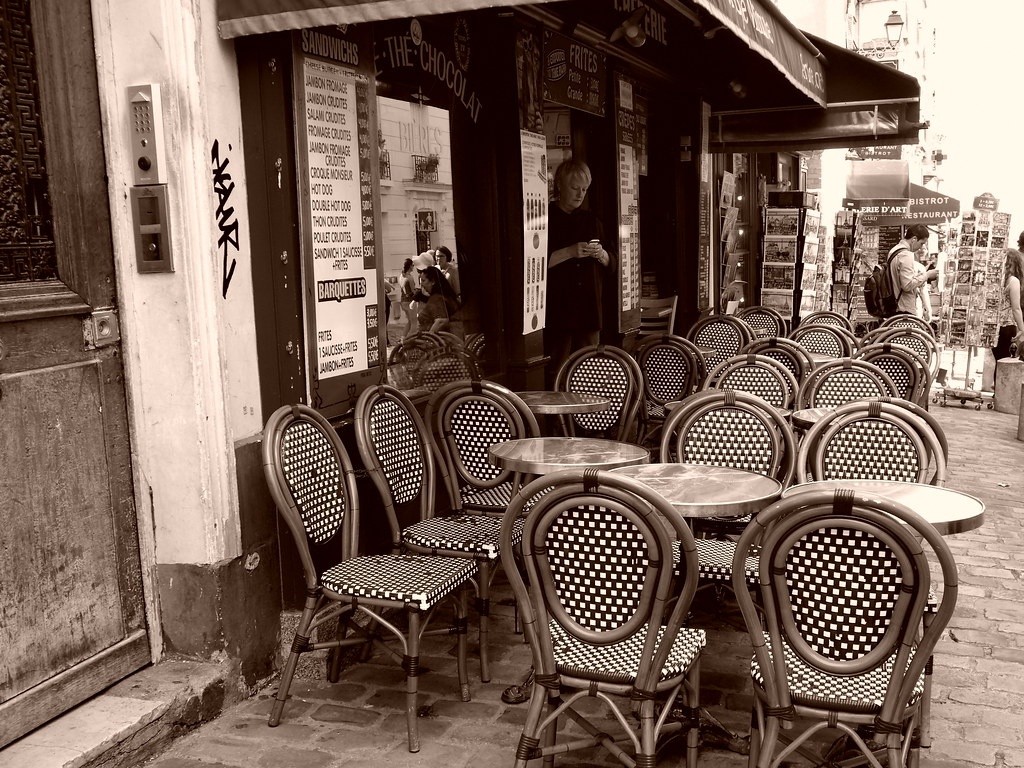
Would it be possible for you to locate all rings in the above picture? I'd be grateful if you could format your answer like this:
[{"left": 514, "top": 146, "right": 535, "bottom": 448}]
[
  {"left": 597, "top": 249, "right": 599, "bottom": 252},
  {"left": 596, "top": 252, "right": 598, "bottom": 255},
  {"left": 582, "top": 248, "right": 587, "bottom": 253}
]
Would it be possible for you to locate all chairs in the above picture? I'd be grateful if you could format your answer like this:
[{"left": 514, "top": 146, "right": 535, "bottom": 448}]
[
  {"left": 686, "top": 307, "right": 940, "bottom": 407},
  {"left": 659, "top": 392, "right": 797, "bottom": 492},
  {"left": 732, "top": 490, "right": 958, "bottom": 768},
  {"left": 424, "top": 379, "right": 555, "bottom": 634},
  {"left": 797, "top": 402, "right": 946, "bottom": 489},
  {"left": 500, "top": 468, "right": 706, "bottom": 767},
  {"left": 630, "top": 334, "right": 706, "bottom": 446},
  {"left": 354, "top": 384, "right": 526, "bottom": 682},
  {"left": 702, "top": 353, "right": 788, "bottom": 409},
  {"left": 638, "top": 296, "right": 678, "bottom": 335},
  {"left": 261, "top": 404, "right": 479, "bottom": 753},
  {"left": 389, "top": 332, "right": 486, "bottom": 385},
  {"left": 797, "top": 359, "right": 899, "bottom": 411},
  {"left": 555, "top": 344, "right": 644, "bottom": 442}
]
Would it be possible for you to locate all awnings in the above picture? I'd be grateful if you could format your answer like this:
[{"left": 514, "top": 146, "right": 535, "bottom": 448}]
[{"left": 214, "top": 0, "right": 830, "bottom": 112}]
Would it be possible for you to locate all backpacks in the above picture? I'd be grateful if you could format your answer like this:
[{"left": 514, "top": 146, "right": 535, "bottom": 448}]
[{"left": 863, "top": 248, "right": 910, "bottom": 317}]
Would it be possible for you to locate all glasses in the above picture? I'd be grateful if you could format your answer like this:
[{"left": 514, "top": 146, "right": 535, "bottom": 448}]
[
  {"left": 436, "top": 255, "right": 445, "bottom": 257},
  {"left": 413, "top": 265, "right": 414, "bottom": 266}
]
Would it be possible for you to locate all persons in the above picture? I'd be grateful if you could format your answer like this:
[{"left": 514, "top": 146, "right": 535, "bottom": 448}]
[
  {"left": 434, "top": 246, "right": 460, "bottom": 298},
  {"left": 888, "top": 224, "right": 938, "bottom": 316},
  {"left": 1018, "top": 341, "right": 1024, "bottom": 362},
  {"left": 543, "top": 158, "right": 611, "bottom": 392},
  {"left": 384, "top": 279, "right": 391, "bottom": 346},
  {"left": 990, "top": 248, "right": 1024, "bottom": 390},
  {"left": 1017, "top": 230, "right": 1024, "bottom": 264},
  {"left": 410, "top": 252, "right": 437, "bottom": 314},
  {"left": 913, "top": 260, "right": 932, "bottom": 324},
  {"left": 418, "top": 265, "right": 461, "bottom": 334},
  {"left": 399, "top": 257, "right": 419, "bottom": 342},
  {"left": 916, "top": 244, "right": 937, "bottom": 288}
]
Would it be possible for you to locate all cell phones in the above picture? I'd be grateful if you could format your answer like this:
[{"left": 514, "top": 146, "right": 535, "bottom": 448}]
[{"left": 584, "top": 239, "right": 600, "bottom": 252}]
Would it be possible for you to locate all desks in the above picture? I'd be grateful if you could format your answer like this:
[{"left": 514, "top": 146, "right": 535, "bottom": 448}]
[
  {"left": 781, "top": 479, "right": 985, "bottom": 537},
  {"left": 665, "top": 401, "right": 790, "bottom": 425},
  {"left": 792, "top": 407, "right": 845, "bottom": 433},
  {"left": 512, "top": 390, "right": 609, "bottom": 415},
  {"left": 488, "top": 437, "right": 651, "bottom": 475},
  {"left": 607, "top": 463, "right": 783, "bottom": 518}
]
